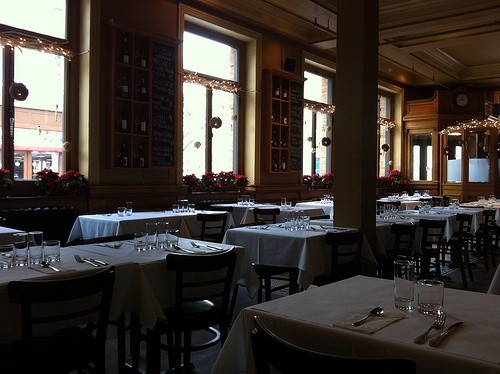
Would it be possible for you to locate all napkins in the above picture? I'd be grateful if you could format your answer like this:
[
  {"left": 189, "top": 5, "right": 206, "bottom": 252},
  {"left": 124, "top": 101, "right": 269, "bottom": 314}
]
[{"left": 334, "top": 304, "right": 405, "bottom": 333}]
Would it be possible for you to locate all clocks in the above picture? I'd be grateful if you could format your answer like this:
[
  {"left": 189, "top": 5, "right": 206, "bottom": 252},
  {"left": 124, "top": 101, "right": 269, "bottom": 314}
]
[{"left": 455, "top": 93, "right": 469, "bottom": 107}]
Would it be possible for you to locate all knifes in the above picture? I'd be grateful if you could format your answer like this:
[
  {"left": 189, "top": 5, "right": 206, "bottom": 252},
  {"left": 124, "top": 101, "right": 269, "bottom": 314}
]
[{"left": 428, "top": 321, "right": 464, "bottom": 347}]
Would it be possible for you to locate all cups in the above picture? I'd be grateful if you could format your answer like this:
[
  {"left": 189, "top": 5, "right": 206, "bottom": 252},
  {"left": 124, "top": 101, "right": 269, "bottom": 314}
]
[
  {"left": 134, "top": 222, "right": 180, "bottom": 252},
  {"left": 417, "top": 279, "right": 444, "bottom": 316},
  {"left": 173, "top": 184, "right": 496, "bottom": 231},
  {"left": 118, "top": 201, "right": 132, "bottom": 216},
  {"left": 393, "top": 260, "right": 416, "bottom": 310},
  {"left": 0, "top": 229, "right": 61, "bottom": 269}
]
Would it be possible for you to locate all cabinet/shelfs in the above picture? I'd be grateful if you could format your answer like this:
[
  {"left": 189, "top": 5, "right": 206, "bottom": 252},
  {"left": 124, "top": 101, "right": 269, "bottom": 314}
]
[
  {"left": 107, "top": 16, "right": 154, "bottom": 172},
  {"left": 264, "top": 67, "right": 297, "bottom": 175}
]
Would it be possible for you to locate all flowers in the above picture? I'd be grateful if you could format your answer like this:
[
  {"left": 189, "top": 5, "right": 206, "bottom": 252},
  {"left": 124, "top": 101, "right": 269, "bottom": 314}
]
[
  {"left": 182, "top": 172, "right": 249, "bottom": 193},
  {"left": 0, "top": 168, "right": 12, "bottom": 198},
  {"left": 376, "top": 170, "right": 403, "bottom": 192},
  {"left": 303, "top": 173, "right": 335, "bottom": 190},
  {"left": 31, "top": 168, "right": 87, "bottom": 195}
]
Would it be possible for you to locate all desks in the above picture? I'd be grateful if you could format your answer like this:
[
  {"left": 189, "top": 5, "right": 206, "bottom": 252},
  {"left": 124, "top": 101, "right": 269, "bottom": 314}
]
[
  {"left": 210, "top": 275, "right": 500, "bottom": 374},
  {"left": 375, "top": 208, "right": 456, "bottom": 257},
  {"left": 210, "top": 202, "right": 325, "bottom": 227},
  {"left": 66, "top": 207, "right": 236, "bottom": 244},
  {"left": 221, "top": 219, "right": 379, "bottom": 294},
  {"left": 295, "top": 199, "right": 334, "bottom": 219},
  {"left": 71, "top": 234, "right": 260, "bottom": 374},
  {"left": 0, "top": 247, "right": 139, "bottom": 374},
  {"left": 434, "top": 206, "right": 485, "bottom": 236},
  {"left": 459, "top": 198, "right": 500, "bottom": 227}
]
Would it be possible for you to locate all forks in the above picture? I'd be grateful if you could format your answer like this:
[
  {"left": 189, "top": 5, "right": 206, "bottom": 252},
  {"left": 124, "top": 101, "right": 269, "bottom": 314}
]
[
  {"left": 413, "top": 316, "right": 445, "bottom": 344},
  {"left": 73, "top": 254, "right": 100, "bottom": 267},
  {"left": 190, "top": 241, "right": 217, "bottom": 251},
  {"left": 94, "top": 242, "right": 123, "bottom": 248}
]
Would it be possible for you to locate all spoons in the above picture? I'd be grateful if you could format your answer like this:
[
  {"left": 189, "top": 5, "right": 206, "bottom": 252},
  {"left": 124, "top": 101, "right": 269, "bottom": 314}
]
[
  {"left": 39, "top": 260, "right": 61, "bottom": 272},
  {"left": 352, "top": 307, "right": 384, "bottom": 328}
]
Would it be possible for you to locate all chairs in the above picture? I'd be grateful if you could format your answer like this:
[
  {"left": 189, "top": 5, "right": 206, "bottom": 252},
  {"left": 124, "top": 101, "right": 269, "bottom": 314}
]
[{"left": 0, "top": 196, "right": 500, "bottom": 374}]
